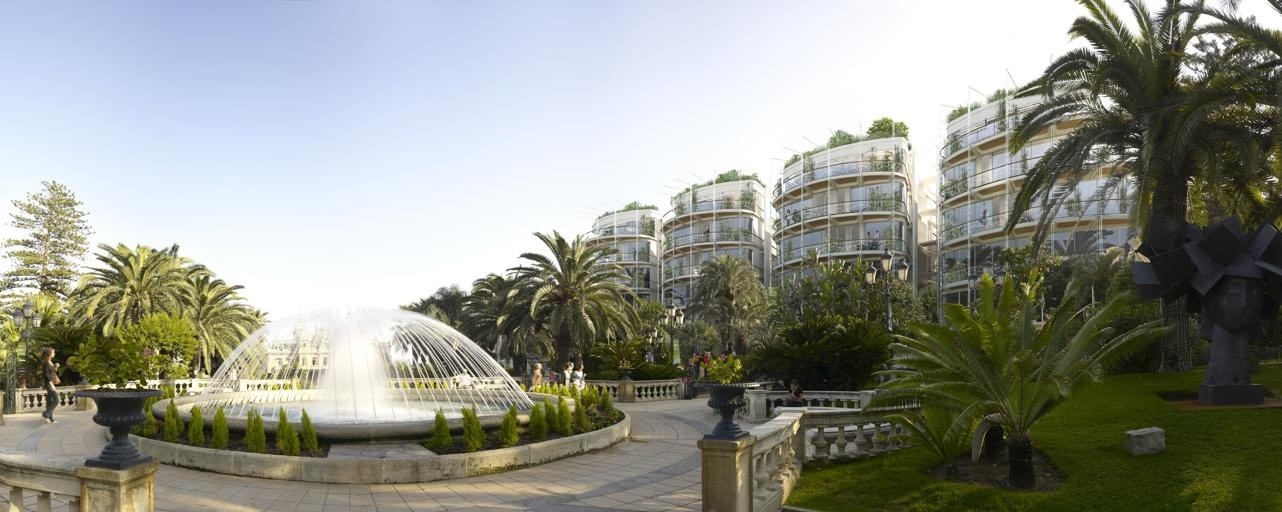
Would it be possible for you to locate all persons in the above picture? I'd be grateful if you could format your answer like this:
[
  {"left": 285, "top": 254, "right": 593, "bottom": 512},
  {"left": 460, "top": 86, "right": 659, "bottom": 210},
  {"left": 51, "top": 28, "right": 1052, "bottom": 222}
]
[
  {"left": 549, "top": 361, "right": 587, "bottom": 391},
  {"left": 979, "top": 208, "right": 989, "bottom": 229},
  {"left": 787, "top": 379, "right": 804, "bottom": 407},
  {"left": 867, "top": 230, "right": 881, "bottom": 249},
  {"left": 531, "top": 362, "right": 543, "bottom": 386},
  {"left": 786, "top": 210, "right": 798, "bottom": 226},
  {"left": 704, "top": 225, "right": 710, "bottom": 242},
  {"left": 40, "top": 347, "right": 61, "bottom": 425}
]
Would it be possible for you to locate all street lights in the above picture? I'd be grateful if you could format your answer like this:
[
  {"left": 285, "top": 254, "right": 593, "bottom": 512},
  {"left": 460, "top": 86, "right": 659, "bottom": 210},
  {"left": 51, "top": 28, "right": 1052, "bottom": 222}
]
[
  {"left": 659, "top": 300, "right": 686, "bottom": 365},
  {"left": 645, "top": 326, "right": 666, "bottom": 366},
  {"left": 967, "top": 255, "right": 1010, "bottom": 310},
  {"left": 10, "top": 299, "right": 43, "bottom": 388},
  {"left": 864, "top": 246, "right": 909, "bottom": 385}
]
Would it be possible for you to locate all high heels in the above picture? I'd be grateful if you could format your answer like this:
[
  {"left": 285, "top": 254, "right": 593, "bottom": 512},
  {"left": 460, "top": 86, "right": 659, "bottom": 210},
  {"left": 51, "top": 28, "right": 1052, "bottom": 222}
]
[{"left": 43, "top": 411, "right": 54, "bottom": 422}]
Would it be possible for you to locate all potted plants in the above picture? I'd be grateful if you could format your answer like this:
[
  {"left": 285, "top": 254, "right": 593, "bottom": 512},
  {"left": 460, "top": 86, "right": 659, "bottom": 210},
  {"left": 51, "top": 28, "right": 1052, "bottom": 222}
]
[
  {"left": 692, "top": 354, "right": 749, "bottom": 441},
  {"left": 67, "top": 331, "right": 164, "bottom": 471},
  {"left": 614, "top": 358, "right": 635, "bottom": 381}
]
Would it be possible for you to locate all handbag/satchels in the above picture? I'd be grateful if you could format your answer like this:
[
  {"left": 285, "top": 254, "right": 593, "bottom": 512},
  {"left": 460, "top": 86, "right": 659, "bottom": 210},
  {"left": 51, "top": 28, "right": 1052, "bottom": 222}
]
[{"left": 54, "top": 372, "right": 60, "bottom": 384}]
